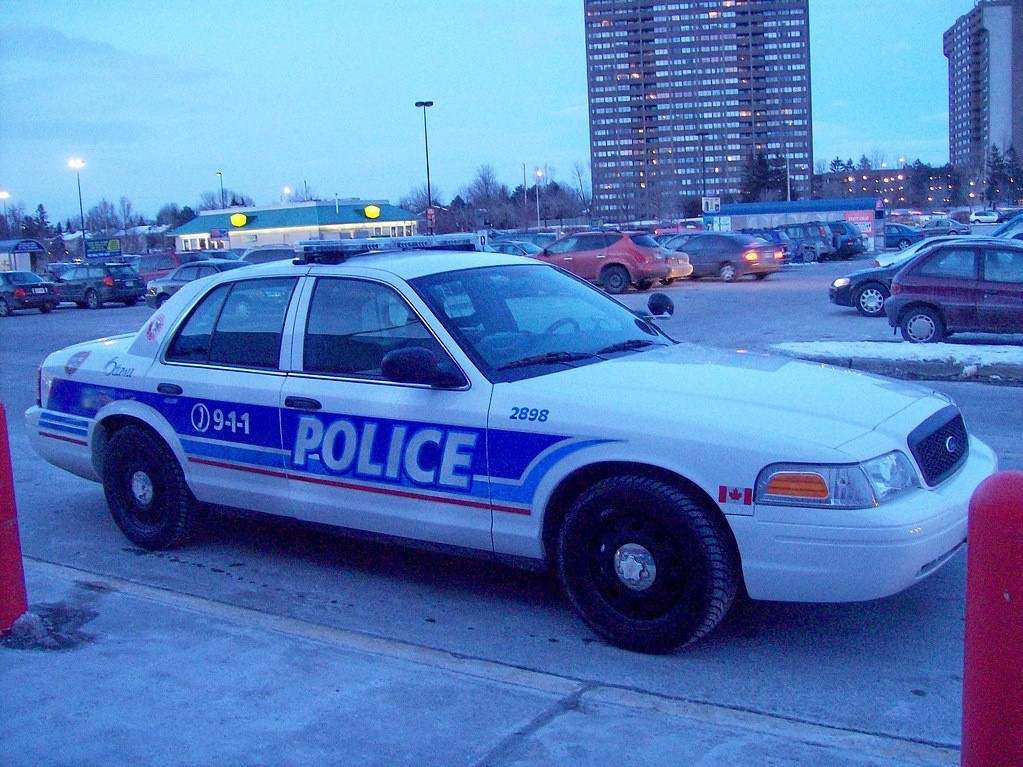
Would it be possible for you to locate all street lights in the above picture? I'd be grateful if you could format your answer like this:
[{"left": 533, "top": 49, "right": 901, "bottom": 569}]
[
  {"left": 74, "top": 159, "right": 88, "bottom": 258},
  {"left": 0, "top": 191, "right": 12, "bottom": 271},
  {"left": 536, "top": 166, "right": 541, "bottom": 227},
  {"left": 216, "top": 171, "right": 224, "bottom": 209},
  {"left": 415, "top": 101, "right": 434, "bottom": 235}
]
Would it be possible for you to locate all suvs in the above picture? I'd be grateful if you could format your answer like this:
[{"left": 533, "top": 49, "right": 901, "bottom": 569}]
[{"left": 123, "top": 249, "right": 214, "bottom": 286}]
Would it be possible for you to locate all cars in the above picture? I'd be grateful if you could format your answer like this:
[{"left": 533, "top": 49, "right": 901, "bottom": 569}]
[
  {"left": 0, "top": 271, "right": 61, "bottom": 317},
  {"left": 201, "top": 243, "right": 297, "bottom": 265},
  {"left": 828, "top": 239, "right": 975, "bottom": 318},
  {"left": 477, "top": 203, "right": 1023, "bottom": 270},
  {"left": 883, "top": 239, "right": 1023, "bottom": 344},
  {"left": 146, "top": 258, "right": 255, "bottom": 310},
  {"left": 671, "top": 232, "right": 785, "bottom": 283},
  {"left": 48, "top": 262, "right": 149, "bottom": 310},
  {"left": 516, "top": 231, "right": 693, "bottom": 295},
  {"left": 872, "top": 235, "right": 1003, "bottom": 270},
  {"left": 22, "top": 235, "right": 1004, "bottom": 655},
  {"left": 79, "top": 255, "right": 141, "bottom": 272}
]
